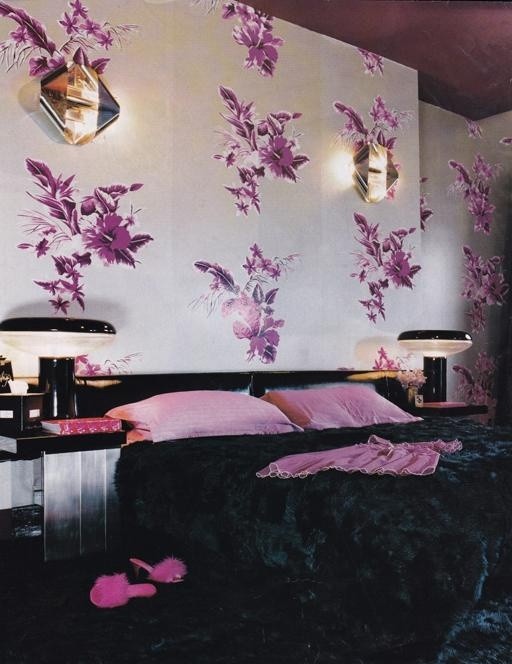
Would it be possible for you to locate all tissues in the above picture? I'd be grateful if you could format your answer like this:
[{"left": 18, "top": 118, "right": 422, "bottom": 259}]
[{"left": 0, "top": 380, "right": 45, "bottom": 430}]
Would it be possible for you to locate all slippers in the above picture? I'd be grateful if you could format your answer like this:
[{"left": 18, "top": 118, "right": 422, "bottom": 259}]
[
  {"left": 130, "top": 556, "right": 188, "bottom": 584},
  {"left": 90, "top": 572, "right": 156, "bottom": 608}
]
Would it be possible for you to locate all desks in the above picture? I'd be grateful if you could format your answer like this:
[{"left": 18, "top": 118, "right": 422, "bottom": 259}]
[
  {"left": 401, "top": 394, "right": 490, "bottom": 421},
  {"left": 2, "top": 418, "right": 135, "bottom": 562}
]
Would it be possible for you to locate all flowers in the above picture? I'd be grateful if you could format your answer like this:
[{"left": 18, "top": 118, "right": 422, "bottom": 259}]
[{"left": 396, "top": 368, "right": 428, "bottom": 390}]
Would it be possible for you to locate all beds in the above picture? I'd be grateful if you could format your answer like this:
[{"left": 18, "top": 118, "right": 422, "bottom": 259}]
[{"left": 74, "top": 364, "right": 510, "bottom": 662}]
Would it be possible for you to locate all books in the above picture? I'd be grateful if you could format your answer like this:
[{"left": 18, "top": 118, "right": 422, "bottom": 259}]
[
  {"left": 40, "top": 417, "right": 123, "bottom": 437},
  {"left": 424, "top": 402, "right": 466, "bottom": 408}
]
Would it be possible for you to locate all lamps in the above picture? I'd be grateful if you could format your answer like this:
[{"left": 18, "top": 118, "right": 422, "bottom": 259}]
[
  {"left": 395, "top": 328, "right": 473, "bottom": 401},
  {"left": 352, "top": 142, "right": 400, "bottom": 203},
  {"left": 39, "top": 61, "right": 122, "bottom": 145},
  {"left": 0, "top": 315, "right": 116, "bottom": 419}
]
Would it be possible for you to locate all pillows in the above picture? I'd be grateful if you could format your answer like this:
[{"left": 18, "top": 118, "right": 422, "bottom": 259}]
[
  {"left": 106, "top": 386, "right": 301, "bottom": 442},
  {"left": 259, "top": 381, "right": 424, "bottom": 432}
]
